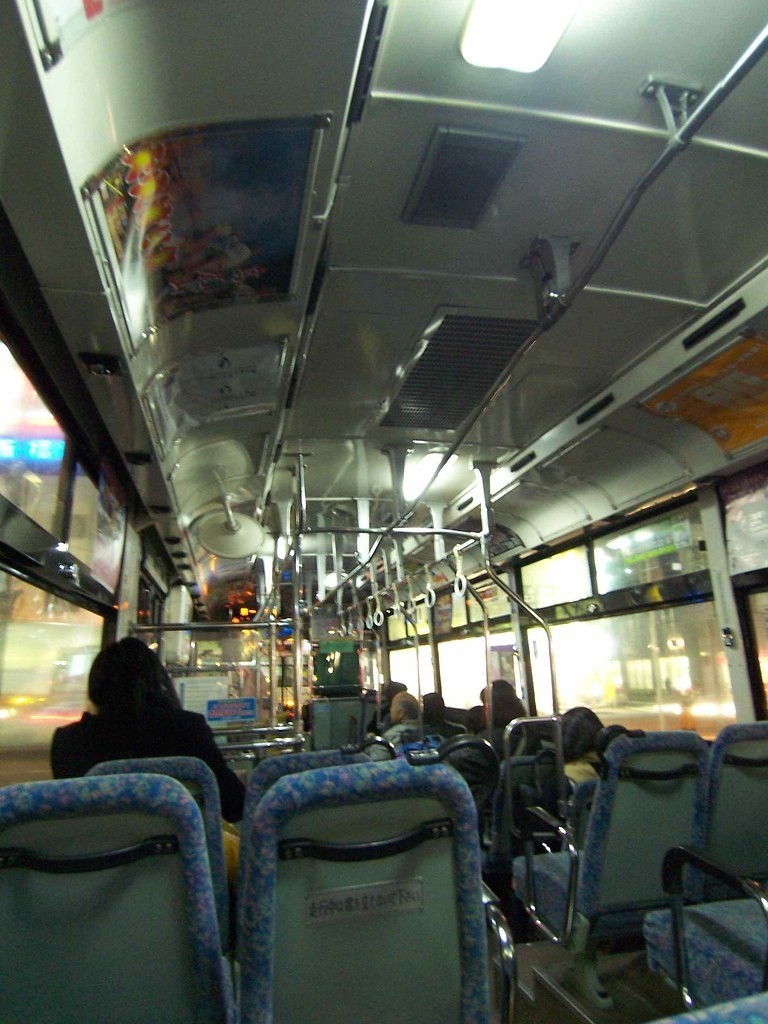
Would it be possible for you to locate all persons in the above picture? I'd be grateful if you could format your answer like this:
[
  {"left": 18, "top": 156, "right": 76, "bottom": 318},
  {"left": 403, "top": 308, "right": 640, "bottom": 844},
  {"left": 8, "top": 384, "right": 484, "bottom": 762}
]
[
  {"left": 364, "top": 679, "right": 633, "bottom": 946},
  {"left": 338, "top": 643, "right": 361, "bottom": 684},
  {"left": 309, "top": 649, "right": 334, "bottom": 686},
  {"left": 49, "top": 633, "right": 247, "bottom": 826}
]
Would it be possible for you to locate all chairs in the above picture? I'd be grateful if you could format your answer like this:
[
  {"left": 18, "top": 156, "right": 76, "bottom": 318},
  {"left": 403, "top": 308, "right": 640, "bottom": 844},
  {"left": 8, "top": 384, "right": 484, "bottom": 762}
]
[{"left": 0, "top": 720, "right": 768, "bottom": 1024}]
